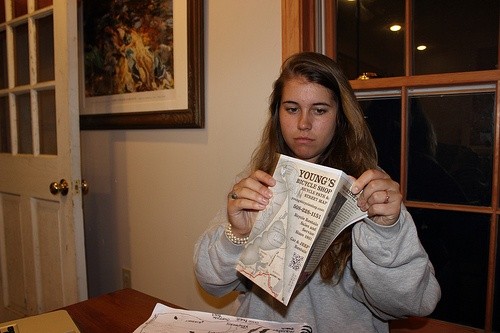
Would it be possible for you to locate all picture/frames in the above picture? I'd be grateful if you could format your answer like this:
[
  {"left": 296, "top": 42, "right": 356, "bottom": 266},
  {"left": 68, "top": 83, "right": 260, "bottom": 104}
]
[{"left": 75, "top": 0, "right": 205, "bottom": 131}]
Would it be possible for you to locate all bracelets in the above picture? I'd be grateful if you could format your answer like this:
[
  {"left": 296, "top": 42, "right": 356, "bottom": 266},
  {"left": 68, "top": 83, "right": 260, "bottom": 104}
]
[{"left": 225, "top": 224, "right": 249, "bottom": 244}]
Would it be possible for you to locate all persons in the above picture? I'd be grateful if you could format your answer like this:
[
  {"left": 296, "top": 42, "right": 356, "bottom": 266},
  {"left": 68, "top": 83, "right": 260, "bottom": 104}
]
[
  {"left": 357, "top": 99, "right": 479, "bottom": 251},
  {"left": 192, "top": 51, "right": 440, "bottom": 333}
]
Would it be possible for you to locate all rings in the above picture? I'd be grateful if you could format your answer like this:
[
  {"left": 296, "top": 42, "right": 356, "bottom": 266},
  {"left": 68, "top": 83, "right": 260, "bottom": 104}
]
[
  {"left": 384, "top": 191, "right": 389, "bottom": 204},
  {"left": 231, "top": 193, "right": 240, "bottom": 199}
]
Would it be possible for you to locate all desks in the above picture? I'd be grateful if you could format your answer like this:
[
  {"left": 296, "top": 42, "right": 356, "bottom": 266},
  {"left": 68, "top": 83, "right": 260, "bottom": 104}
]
[{"left": 0, "top": 287, "right": 189, "bottom": 333}]
